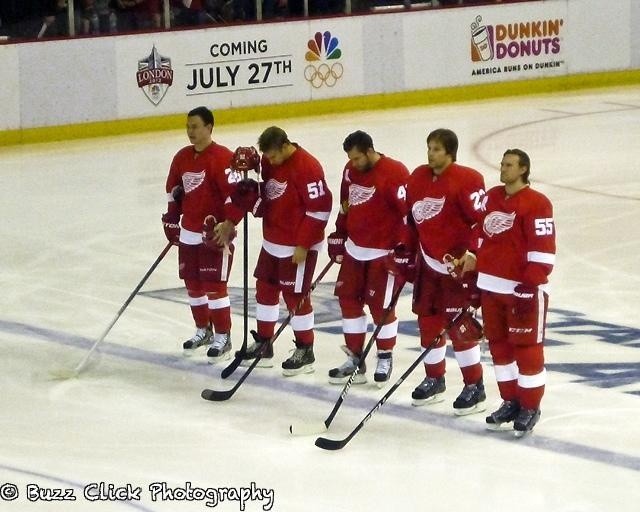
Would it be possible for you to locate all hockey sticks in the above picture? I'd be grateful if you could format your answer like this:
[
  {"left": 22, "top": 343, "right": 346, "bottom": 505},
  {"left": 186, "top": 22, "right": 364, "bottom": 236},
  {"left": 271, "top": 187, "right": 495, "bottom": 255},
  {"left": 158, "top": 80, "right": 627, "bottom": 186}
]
[
  {"left": 290, "top": 276, "right": 407, "bottom": 435},
  {"left": 314, "top": 299, "right": 470, "bottom": 450},
  {"left": 46, "top": 236, "right": 179, "bottom": 379},
  {"left": 201, "top": 255, "right": 336, "bottom": 401},
  {"left": 221, "top": 170, "right": 247, "bottom": 380}
]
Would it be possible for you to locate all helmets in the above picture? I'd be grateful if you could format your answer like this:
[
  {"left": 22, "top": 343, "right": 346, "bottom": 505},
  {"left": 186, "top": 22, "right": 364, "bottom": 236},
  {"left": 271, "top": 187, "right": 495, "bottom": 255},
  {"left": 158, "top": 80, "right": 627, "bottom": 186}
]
[{"left": 232, "top": 146, "right": 260, "bottom": 171}]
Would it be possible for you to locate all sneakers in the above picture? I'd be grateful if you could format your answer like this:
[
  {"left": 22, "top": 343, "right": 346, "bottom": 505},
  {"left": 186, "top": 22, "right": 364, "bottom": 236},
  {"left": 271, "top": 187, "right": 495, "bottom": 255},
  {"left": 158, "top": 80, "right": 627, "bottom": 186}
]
[
  {"left": 183, "top": 326, "right": 214, "bottom": 350},
  {"left": 282, "top": 340, "right": 315, "bottom": 369},
  {"left": 412, "top": 376, "right": 446, "bottom": 399},
  {"left": 453, "top": 384, "right": 486, "bottom": 408},
  {"left": 329, "top": 346, "right": 366, "bottom": 378},
  {"left": 374, "top": 353, "right": 392, "bottom": 381},
  {"left": 486, "top": 401, "right": 520, "bottom": 423},
  {"left": 207, "top": 333, "right": 232, "bottom": 357},
  {"left": 235, "top": 330, "right": 273, "bottom": 360},
  {"left": 514, "top": 409, "right": 541, "bottom": 431}
]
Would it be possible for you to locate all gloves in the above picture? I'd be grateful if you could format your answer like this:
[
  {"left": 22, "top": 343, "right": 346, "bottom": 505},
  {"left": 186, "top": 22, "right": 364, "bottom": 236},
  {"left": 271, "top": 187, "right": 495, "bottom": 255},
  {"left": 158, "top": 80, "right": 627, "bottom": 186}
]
[
  {"left": 456, "top": 312, "right": 483, "bottom": 340},
  {"left": 234, "top": 194, "right": 264, "bottom": 217},
  {"left": 237, "top": 179, "right": 264, "bottom": 194},
  {"left": 515, "top": 285, "right": 538, "bottom": 311},
  {"left": 405, "top": 264, "right": 416, "bottom": 283},
  {"left": 464, "top": 271, "right": 482, "bottom": 309},
  {"left": 394, "top": 244, "right": 411, "bottom": 272},
  {"left": 327, "top": 232, "right": 344, "bottom": 264},
  {"left": 162, "top": 212, "right": 180, "bottom": 246},
  {"left": 202, "top": 215, "right": 225, "bottom": 252}
]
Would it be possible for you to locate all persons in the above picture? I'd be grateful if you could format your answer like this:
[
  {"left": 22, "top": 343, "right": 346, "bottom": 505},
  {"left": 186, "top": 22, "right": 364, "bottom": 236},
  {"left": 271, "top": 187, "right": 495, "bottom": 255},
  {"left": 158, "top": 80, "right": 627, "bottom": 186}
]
[
  {"left": 238, "top": 124, "right": 333, "bottom": 370},
  {"left": 326, "top": 130, "right": 414, "bottom": 383},
  {"left": 1, "top": 2, "right": 371, "bottom": 39},
  {"left": 402, "top": 128, "right": 488, "bottom": 410},
  {"left": 467, "top": 148, "right": 557, "bottom": 431},
  {"left": 160, "top": 106, "right": 246, "bottom": 360}
]
